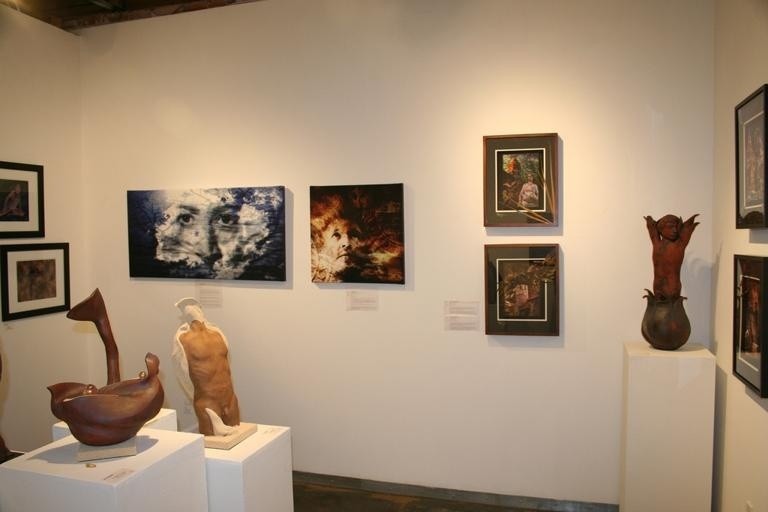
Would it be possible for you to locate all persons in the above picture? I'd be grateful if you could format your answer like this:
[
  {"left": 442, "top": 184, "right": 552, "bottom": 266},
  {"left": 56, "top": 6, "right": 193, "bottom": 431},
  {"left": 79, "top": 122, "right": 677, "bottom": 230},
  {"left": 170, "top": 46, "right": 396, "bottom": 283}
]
[
  {"left": 518, "top": 174, "right": 540, "bottom": 210},
  {"left": 155, "top": 190, "right": 243, "bottom": 280},
  {"left": 643, "top": 214, "right": 700, "bottom": 299},
  {"left": 170, "top": 297, "right": 240, "bottom": 436},
  {"left": 323, "top": 218, "right": 361, "bottom": 282}
]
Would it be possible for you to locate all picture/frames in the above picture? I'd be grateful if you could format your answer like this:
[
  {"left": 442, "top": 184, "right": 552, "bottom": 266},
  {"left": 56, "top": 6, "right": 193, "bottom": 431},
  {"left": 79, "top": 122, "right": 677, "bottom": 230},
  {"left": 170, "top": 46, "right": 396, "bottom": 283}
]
[
  {"left": 733, "top": 83, "right": 768, "bottom": 229},
  {"left": 0, "top": 160, "right": 47, "bottom": 239},
  {"left": 732, "top": 253, "right": 767, "bottom": 398},
  {"left": 483, "top": 242, "right": 560, "bottom": 337},
  {"left": 0, "top": 243, "right": 68, "bottom": 322},
  {"left": 482, "top": 132, "right": 559, "bottom": 228}
]
